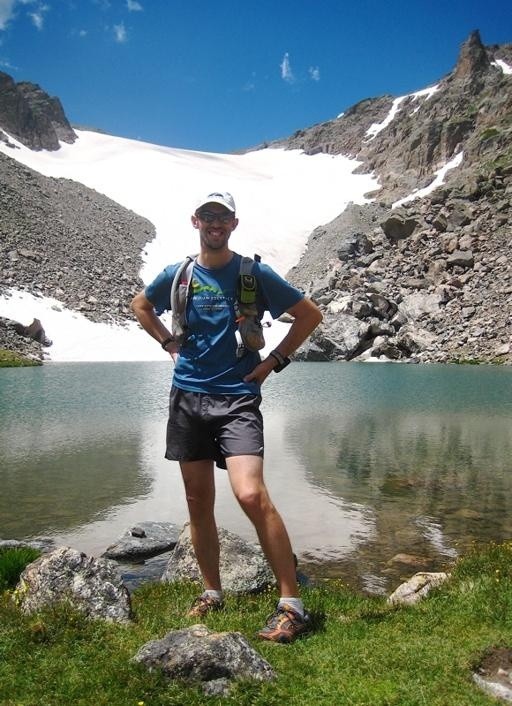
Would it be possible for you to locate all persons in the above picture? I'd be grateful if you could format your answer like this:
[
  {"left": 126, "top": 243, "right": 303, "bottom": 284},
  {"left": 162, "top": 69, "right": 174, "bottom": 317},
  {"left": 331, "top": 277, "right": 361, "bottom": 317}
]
[{"left": 130, "top": 189, "right": 327, "bottom": 647}]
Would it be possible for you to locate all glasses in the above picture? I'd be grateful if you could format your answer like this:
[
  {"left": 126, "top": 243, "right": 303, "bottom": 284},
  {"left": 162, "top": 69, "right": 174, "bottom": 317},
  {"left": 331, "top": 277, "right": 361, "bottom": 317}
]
[{"left": 196, "top": 210, "right": 234, "bottom": 223}]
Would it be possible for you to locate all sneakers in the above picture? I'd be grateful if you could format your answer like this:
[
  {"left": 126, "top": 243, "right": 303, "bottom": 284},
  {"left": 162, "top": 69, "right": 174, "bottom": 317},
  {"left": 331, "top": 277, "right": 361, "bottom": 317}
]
[
  {"left": 258, "top": 601, "right": 313, "bottom": 643},
  {"left": 183, "top": 592, "right": 226, "bottom": 618}
]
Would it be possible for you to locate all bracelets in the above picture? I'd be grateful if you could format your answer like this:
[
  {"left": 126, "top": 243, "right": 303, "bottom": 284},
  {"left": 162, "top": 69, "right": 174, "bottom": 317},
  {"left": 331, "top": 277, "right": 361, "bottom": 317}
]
[
  {"left": 268, "top": 349, "right": 291, "bottom": 372},
  {"left": 162, "top": 336, "right": 175, "bottom": 352}
]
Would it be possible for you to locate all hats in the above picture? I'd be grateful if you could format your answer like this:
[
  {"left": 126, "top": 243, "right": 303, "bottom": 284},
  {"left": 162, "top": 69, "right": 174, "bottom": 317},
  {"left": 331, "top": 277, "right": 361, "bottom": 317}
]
[{"left": 193, "top": 190, "right": 238, "bottom": 214}]
